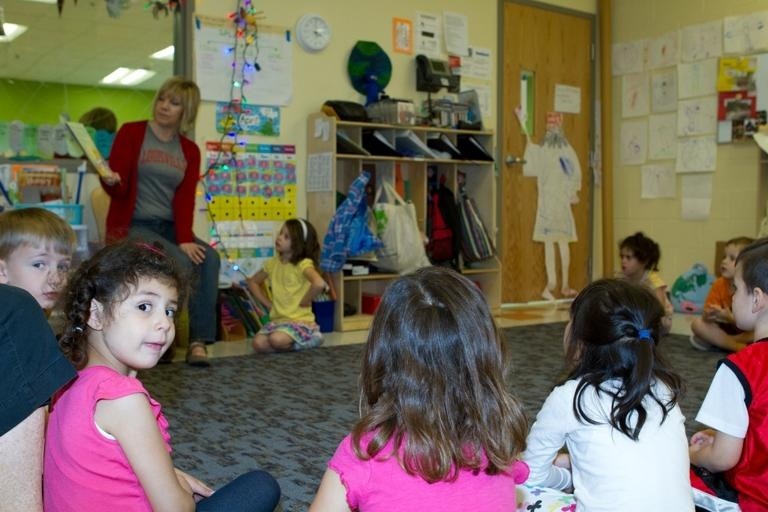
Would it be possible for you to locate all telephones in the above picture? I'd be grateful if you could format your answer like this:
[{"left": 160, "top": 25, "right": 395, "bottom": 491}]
[{"left": 416, "top": 54, "right": 457, "bottom": 91}]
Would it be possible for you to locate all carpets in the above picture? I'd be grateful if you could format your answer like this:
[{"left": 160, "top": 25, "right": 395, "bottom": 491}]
[{"left": 139, "top": 320, "right": 727, "bottom": 512}]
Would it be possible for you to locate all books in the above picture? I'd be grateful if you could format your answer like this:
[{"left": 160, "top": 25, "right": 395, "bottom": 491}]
[
  {"left": 425, "top": 98, "right": 470, "bottom": 128},
  {"left": 335, "top": 129, "right": 494, "bottom": 161}
]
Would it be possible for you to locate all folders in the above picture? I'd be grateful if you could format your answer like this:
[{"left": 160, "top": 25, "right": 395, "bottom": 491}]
[{"left": 362, "top": 129, "right": 493, "bottom": 161}]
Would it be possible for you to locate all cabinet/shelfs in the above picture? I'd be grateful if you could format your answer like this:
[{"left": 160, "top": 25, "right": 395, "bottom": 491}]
[{"left": 306, "top": 111, "right": 502, "bottom": 333}]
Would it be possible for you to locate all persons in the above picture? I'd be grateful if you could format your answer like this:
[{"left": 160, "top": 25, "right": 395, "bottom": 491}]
[
  {"left": 618, "top": 232, "right": 674, "bottom": 336},
  {"left": 98, "top": 76, "right": 220, "bottom": 365},
  {"left": 514, "top": 279, "right": 695, "bottom": 512},
  {"left": 43, "top": 240, "right": 281, "bottom": 512},
  {"left": 688, "top": 235, "right": 755, "bottom": 353},
  {"left": 0, "top": 207, "right": 77, "bottom": 310},
  {"left": 247, "top": 218, "right": 325, "bottom": 353},
  {"left": 684, "top": 237, "right": 767, "bottom": 512},
  {"left": 0, "top": 284, "right": 78, "bottom": 512},
  {"left": 306, "top": 266, "right": 529, "bottom": 512}
]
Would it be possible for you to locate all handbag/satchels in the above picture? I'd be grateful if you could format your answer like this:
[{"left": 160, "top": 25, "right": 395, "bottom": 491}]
[
  {"left": 669, "top": 263, "right": 716, "bottom": 314},
  {"left": 345, "top": 177, "right": 495, "bottom": 278}
]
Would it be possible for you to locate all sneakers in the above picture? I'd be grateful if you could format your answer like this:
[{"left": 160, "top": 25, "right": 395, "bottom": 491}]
[
  {"left": 292, "top": 331, "right": 324, "bottom": 351},
  {"left": 159, "top": 341, "right": 176, "bottom": 359},
  {"left": 186, "top": 342, "right": 210, "bottom": 367},
  {"left": 689, "top": 333, "right": 712, "bottom": 352}
]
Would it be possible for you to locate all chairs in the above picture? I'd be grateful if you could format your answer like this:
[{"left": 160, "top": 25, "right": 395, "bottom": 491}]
[{"left": 89, "top": 186, "right": 111, "bottom": 252}]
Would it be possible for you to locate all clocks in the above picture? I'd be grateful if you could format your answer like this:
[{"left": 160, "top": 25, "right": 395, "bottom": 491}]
[{"left": 295, "top": 14, "right": 331, "bottom": 54}]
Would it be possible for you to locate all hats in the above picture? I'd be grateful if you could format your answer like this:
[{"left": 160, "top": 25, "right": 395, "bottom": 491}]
[{"left": 348, "top": 40, "right": 392, "bottom": 96}]
